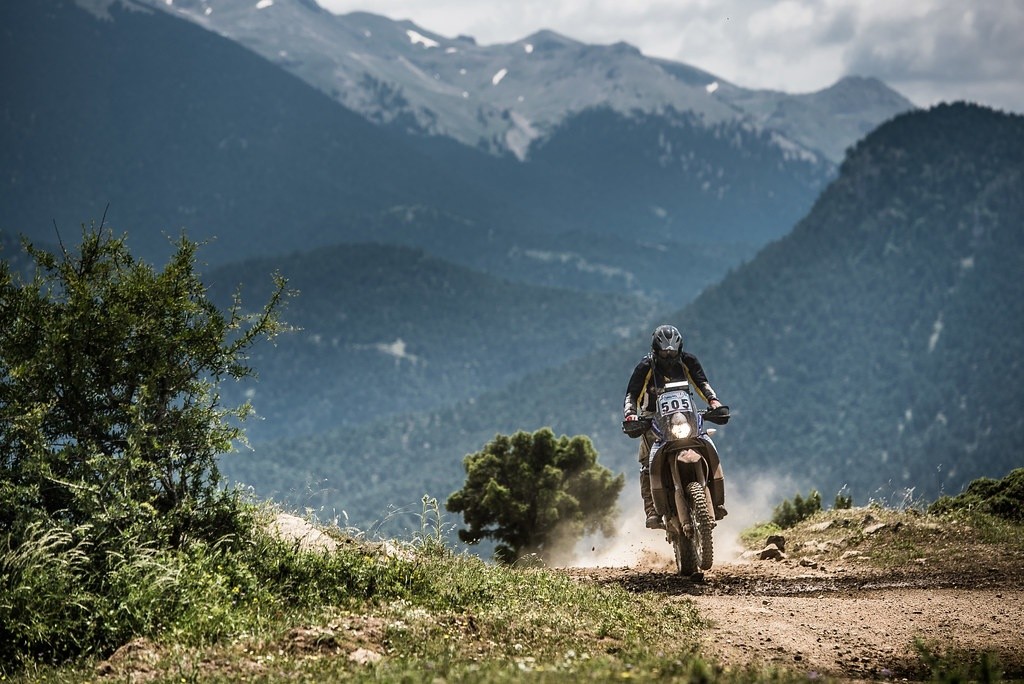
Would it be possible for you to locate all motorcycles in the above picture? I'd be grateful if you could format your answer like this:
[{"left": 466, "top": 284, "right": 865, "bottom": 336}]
[{"left": 621, "top": 391, "right": 732, "bottom": 579}]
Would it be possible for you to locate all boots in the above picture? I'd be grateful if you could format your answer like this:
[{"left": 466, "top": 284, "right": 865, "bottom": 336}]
[
  {"left": 639, "top": 468, "right": 663, "bottom": 527},
  {"left": 715, "top": 504, "right": 727, "bottom": 520}
]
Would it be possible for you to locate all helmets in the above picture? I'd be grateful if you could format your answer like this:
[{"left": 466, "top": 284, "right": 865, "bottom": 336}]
[{"left": 650, "top": 324, "right": 684, "bottom": 353}]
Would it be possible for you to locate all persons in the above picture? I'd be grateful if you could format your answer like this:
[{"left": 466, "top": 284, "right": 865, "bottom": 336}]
[{"left": 624, "top": 325, "right": 728, "bottom": 528}]
